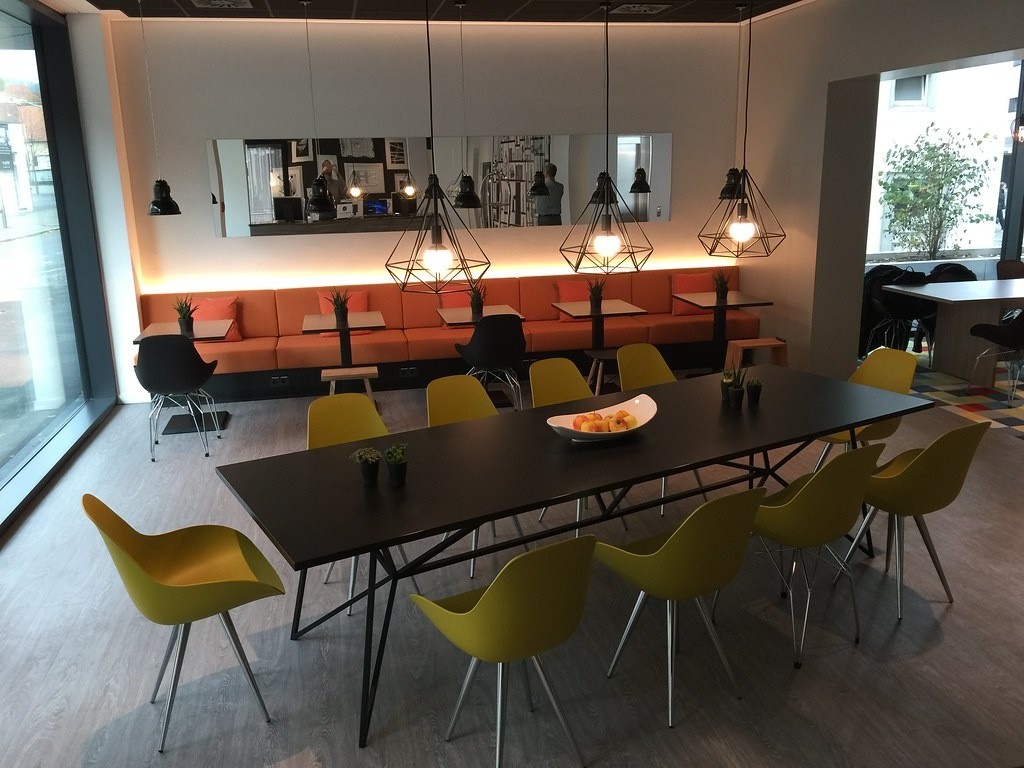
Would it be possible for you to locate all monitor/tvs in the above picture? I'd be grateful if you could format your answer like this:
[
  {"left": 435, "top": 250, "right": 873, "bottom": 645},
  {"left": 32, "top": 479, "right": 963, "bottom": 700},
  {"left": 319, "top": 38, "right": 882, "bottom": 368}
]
[
  {"left": 392, "top": 192, "right": 417, "bottom": 213},
  {"left": 273, "top": 197, "right": 304, "bottom": 220}
]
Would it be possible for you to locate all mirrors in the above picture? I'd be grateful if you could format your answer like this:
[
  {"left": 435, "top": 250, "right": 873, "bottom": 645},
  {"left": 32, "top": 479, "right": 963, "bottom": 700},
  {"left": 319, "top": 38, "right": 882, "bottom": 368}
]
[
  {"left": 395, "top": 169, "right": 423, "bottom": 201},
  {"left": 201, "top": 132, "right": 680, "bottom": 241}
]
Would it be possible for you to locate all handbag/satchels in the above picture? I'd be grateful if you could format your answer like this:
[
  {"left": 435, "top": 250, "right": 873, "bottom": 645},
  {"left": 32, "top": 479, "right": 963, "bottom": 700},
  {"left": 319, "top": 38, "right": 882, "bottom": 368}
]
[{"left": 878, "top": 266, "right": 930, "bottom": 320}]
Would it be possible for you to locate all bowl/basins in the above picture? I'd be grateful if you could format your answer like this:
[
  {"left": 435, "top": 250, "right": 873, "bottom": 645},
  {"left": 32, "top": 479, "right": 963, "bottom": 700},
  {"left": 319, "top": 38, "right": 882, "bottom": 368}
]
[{"left": 547, "top": 394, "right": 657, "bottom": 443}]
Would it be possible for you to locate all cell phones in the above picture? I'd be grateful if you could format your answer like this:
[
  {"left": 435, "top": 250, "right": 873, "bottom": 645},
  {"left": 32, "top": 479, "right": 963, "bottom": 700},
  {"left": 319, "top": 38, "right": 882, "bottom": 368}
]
[{"left": 327, "top": 165, "right": 336, "bottom": 170}]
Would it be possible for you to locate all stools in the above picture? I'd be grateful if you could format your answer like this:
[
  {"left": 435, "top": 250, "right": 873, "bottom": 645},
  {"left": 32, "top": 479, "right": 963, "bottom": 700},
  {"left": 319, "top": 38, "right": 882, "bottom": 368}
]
[
  {"left": 583, "top": 341, "right": 620, "bottom": 395},
  {"left": 320, "top": 362, "right": 380, "bottom": 412},
  {"left": 724, "top": 336, "right": 795, "bottom": 371}
]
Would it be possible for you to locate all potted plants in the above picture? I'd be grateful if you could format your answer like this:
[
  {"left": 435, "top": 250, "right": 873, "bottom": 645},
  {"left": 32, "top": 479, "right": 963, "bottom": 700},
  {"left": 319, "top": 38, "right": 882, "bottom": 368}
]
[
  {"left": 171, "top": 290, "right": 202, "bottom": 330},
  {"left": 317, "top": 286, "right": 354, "bottom": 323},
  {"left": 583, "top": 273, "right": 612, "bottom": 308},
  {"left": 725, "top": 362, "right": 749, "bottom": 405},
  {"left": 744, "top": 376, "right": 763, "bottom": 405},
  {"left": 719, "top": 369, "right": 735, "bottom": 404},
  {"left": 707, "top": 268, "right": 732, "bottom": 304},
  {"left": 385, "top": 439, "right": 411, "bottom": 488},
  {"left": 347, "top": 442, "right": 383, "bottom": 487},
  {"left": 466, "top": 281, "right": 491, "bottom": 315}
]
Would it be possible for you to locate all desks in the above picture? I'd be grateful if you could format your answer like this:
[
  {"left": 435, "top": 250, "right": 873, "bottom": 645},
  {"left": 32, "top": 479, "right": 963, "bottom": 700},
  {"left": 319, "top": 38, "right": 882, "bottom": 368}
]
[
  {"left": 300, "top": 306, "right": 388, "bottom": 416},
  {"left": 248, "top": 209, "right": 440, "bottom": 236},
  {"left": 879, "top": 279, "right": 1024, "bottom": 390},
  {"left": 673, "top": 283, "right": 780, "bottom": 380},
  {"left": 131, "top": 316, "right": 235, "bottom": 437},
  {"left": 551, "top": 295, "right": 650, "bottom": 400},
  {"left": 437, "top": 297, "right": 531, "bottom": 409},
  {"left": 212, "top": 356, "right": 949, "bottom": 752}
]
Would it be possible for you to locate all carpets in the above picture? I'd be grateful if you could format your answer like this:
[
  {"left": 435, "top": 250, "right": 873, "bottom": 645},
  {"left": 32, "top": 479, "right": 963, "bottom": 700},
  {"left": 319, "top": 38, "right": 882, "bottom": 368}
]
[{"left": 879, "top": 350, "right": 1024, "bottom": 440}]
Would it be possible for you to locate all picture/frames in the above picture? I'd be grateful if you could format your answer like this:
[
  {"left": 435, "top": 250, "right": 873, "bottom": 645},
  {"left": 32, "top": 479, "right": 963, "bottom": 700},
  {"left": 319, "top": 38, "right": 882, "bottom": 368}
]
[
  {"left": 290, "top": 139, "right": 314, "bottom": 163},
  {"left": 343, "top": 162, "right": 385, "bottom": 195},
  {"left": 384, "top": 137, "right": 409, "bottom": 171}
]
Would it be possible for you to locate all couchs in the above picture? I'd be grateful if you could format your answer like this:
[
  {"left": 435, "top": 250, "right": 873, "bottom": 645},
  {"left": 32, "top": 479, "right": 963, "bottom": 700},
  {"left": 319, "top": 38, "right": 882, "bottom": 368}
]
[{"left": 132, "top": 267, "right": 766, "bottom": 403}]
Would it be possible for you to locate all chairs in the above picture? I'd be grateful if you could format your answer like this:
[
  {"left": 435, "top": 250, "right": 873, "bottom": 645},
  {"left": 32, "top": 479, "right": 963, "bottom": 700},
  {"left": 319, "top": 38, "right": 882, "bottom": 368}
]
[
  {"left": 612, "top": 342, "right": 710, "bottom": 518},
  {"left": 527, "top": 355, "right": 634, "bottom": 539},
  {"left": 453, "top": 313, "right": 528, "bottom": 412},
  {"left": 996, "top": 260, "right": 1024, "bottom": 280},
  {"left": 300, "top": 391, "right": 425, "bottom": 617},
  {"left": 829, "top": 415, "right": 993, "bottom": 623},
  {"left": 78, "top": 491, "right": 292, "bottom": 759},
  {"left": 961, "top": 305, "right": 1024, "bottom": 408},
  {"left": 421, "top": 371, "right": 534, "bottom": 581},
  {"left": 810, "top": 347, "right": 918, "bottom": 474},
  {"left": 705, "top": 439, "right": 889, "bottom": 674},
  {"left": 406, "top": 530, "right": 599, "bottom": 768},
  {"left": 862, "top": 264, "right": 932, "bottom": 368},
  {"left": 133, "top": 330, "right": 221, "bottom": 465},
  {"left": 595, "top": 483, "right": 770, "bottom": 730},
  {"left": 929, "top": 261, "right": 979, "bottom": 284}
]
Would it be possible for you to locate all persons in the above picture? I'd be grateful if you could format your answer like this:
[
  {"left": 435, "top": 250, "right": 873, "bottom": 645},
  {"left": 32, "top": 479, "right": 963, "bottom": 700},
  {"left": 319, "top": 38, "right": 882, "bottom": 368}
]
[
  {"left": 318, "top": 160, "right": 346, "bottom": 199},
  {"left": 535, "top": 163, "right": 565, "bottom": 226}
]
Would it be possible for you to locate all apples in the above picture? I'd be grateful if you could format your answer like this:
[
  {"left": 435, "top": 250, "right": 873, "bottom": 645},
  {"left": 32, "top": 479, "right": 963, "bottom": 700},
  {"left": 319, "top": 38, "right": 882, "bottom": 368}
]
[{"left": 573, "top": 410, "right": 637, "bottom": 432}]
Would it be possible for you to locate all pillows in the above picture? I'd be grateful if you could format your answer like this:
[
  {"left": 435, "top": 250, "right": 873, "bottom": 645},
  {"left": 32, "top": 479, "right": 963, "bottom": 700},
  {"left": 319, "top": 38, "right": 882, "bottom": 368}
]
[
  {"left": 183, "top": 292, "right": 242, "bottom": 345},
  {"left": 555, "top": 280, "right": 608, "bottom": 324},
  {"left": 440, "top": 280, "right": 490, "bottom": 331},
  {"left": 668, "top": 271, "right": 715, "bottom": 317},
  {"left": 314, "top": 287, "right": 372, "bottom": 336}
]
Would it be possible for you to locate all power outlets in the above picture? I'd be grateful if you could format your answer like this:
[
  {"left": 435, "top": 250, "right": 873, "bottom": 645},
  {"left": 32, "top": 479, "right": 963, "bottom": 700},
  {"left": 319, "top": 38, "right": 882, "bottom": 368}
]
[
  {"left": 399, "top": 367, "right": 408, "bottom": 378},
  {"left": 270, "top": 377, "right": 279, "bottom": 387},
  {"left": 522, "top": 360, "right": 529, "bottom": 368},
  {"left": 530, "top": 359, "right": 539, "bottom": 364},
  {"left": 408, "top": 367, "right": 418, "bottom": 378},
  {"left": 280, "top": 376, "right": 289, "bottom": 387}
]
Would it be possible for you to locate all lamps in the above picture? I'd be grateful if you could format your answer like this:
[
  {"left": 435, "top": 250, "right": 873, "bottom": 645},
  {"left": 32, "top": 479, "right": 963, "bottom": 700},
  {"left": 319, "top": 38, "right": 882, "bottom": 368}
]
[
  {"left": 446, "top": 168, "right": 474, "bottom": 197},
  {"left": 132, "top": 0, "right": 184, "bottom": 218},
  {"left": 554, "top": 5, "right": 656, "bottom": 279},
  {"left": 453, "top": 3, "right": 490, "bottom": 213},
  {"left": 451, "top": 172, "right": 482, "bottom": 210},
  {"left": 589, "top": 31, "right": 623, "bottom": 206},
  {"left": 381, "top": 0, "right": 493, "bottom": 297},
  {"left": 628, "top": 164, "right": 653, "bottom": 196},
  {"left": 342, "top": 167, "right": 370, "bottom": 200},
  {"left": 718, "top": 4, "right": 752, "bottom": 201},
  {"left": 424, "top": 172, "right": 446, "bottom": 199},
  {"left": 696, "top": 9, "right": 790, "bottom": 262},
  {"left": 298, "top": 1, "right": 337, "bottom": 214},
  {"left": 526, "top": 167, "right": 552, "bottom": 198}
]
